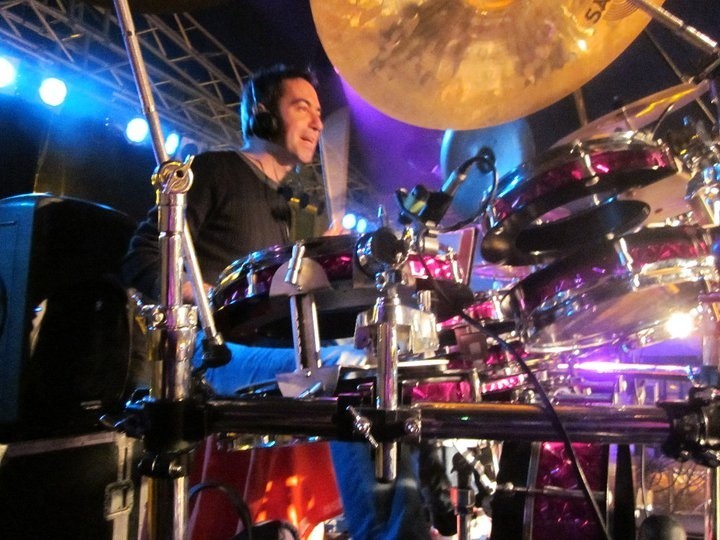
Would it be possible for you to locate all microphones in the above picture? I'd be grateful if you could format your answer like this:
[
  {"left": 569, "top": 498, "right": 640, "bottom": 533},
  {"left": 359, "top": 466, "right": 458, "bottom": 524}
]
[{"left": 416, "top": 158, "right": 473, "bottom": 229}]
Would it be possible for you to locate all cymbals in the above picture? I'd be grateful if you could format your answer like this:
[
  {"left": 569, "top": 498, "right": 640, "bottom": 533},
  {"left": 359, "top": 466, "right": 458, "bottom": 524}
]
[
  {"left": 310, "top": 1, "right": 666, "bottom": 130},
  {"left": 548, "top": 79, "right": 710, "bottom": 149},
  {"left": 472, "top": 258, "right": 542, "bottom": 281}
]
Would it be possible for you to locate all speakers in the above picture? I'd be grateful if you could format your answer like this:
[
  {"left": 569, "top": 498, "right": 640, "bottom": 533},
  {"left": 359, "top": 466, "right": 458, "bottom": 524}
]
[{"left": 0, "top": 192, "right": 139, "bottom": 439}]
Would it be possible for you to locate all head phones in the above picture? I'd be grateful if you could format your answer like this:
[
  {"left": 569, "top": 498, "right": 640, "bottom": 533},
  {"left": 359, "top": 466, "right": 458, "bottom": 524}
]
[{"left": 250, "top": 67, "right": 278, "bottom": 139}]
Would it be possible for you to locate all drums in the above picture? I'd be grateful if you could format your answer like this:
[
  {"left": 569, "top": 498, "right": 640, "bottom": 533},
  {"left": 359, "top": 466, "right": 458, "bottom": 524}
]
[
  {"left": 509, "top": 223, "right": 716, "bottom": 354},
  {"left": 615, "top": 143, "right": 693, "bottom": 224},
  {"left": 207, "top": 229, "right": 464, "bottom": 349},
  {"left": 443, "top": 329, "right": 531, "bottom": 373},
  {"left": 572, "top": 361, "right": 700, "bottom": 410},
  {"left": 478, "top": 131, "right": 679, "bottom": 241},
  {"left": 436, "top": 289, "right": 511, "bottom": 331},
  {"left": 357, "top": 374, "right": 481, "bottom": 502},
  {"left": 524, "top": 387, "right": 632, "bottom": 540}
]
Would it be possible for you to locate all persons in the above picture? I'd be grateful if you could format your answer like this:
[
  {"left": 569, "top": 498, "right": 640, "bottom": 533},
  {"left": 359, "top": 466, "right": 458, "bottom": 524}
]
[
  {"left": 638, "top": 514, "right": 686, "bottom": 540},
  {"left": 125, "top": 67, "right": 436, "bottom": 540}
]
